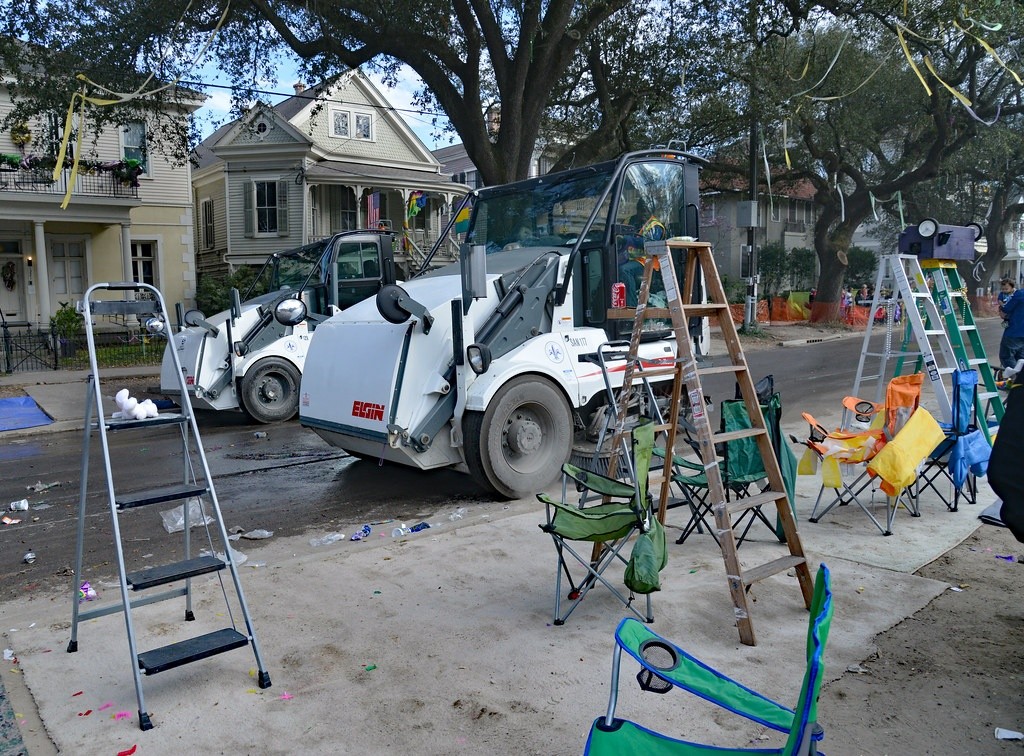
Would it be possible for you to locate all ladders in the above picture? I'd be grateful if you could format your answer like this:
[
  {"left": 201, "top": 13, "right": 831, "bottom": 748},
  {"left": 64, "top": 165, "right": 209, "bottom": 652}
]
[
  {"left": 895, "top": 258, "right": 1006, "bottom": 446},
  {"left": 845, "top": 254, "right": 983, "bottom": 433},
  {"left": 67, "top": 281, "right": 273, "bottom": 731},
  {"left": 585, "top": 239, "right": 817, "bottom": 647}
]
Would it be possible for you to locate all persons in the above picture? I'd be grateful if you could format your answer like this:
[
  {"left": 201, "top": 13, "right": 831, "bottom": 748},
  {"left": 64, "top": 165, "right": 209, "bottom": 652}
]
[
  {"left": 987, "top": 278, "right": 1024, "bottom": 544},
  {"left": 810, "top": 285, "right": 902, "bottom": 327},
  {"left": 618, "top": 198, "right": 667, "bottom": 308}
]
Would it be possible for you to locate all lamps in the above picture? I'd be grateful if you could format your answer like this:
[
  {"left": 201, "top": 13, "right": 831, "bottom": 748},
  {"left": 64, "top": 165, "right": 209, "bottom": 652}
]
[{"left": 28, "top": 260, "right": 32, "bottom": 267}]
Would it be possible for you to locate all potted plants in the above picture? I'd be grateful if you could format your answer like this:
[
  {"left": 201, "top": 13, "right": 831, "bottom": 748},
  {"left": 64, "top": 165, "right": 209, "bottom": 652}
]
[{"left": 55, "top": 305, "right": 83, "bottom": 359}]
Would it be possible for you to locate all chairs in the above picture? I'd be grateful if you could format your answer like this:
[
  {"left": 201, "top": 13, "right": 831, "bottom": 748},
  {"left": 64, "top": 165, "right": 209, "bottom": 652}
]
[
  {"left": 534, "top": 360, "right": 1012, "bottom": 627},
  {"left": 578, "top": 561, "right": 835, "bottom": 756}
]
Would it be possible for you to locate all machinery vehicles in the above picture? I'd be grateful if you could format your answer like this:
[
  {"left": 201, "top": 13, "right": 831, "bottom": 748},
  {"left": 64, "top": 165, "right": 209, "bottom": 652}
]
[{"left": 157, "top": 138, "right": 714, "bottom": 500}]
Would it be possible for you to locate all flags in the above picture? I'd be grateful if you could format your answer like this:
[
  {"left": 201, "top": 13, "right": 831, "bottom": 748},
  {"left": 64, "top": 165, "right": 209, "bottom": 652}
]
[{"left": 368, "top": 191, "right": 381, "bottom": 231}]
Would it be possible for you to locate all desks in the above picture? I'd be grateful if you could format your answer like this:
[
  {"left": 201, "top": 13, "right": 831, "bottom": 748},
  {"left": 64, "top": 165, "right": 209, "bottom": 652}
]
[{"left": 858, "top": 298, "right": 904, "bottom": 306}]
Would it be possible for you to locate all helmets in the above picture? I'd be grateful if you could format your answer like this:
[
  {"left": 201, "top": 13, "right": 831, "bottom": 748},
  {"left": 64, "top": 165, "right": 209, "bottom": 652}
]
[{"left": 638, "top": 196, "right": 655, "bottom": 210}]
